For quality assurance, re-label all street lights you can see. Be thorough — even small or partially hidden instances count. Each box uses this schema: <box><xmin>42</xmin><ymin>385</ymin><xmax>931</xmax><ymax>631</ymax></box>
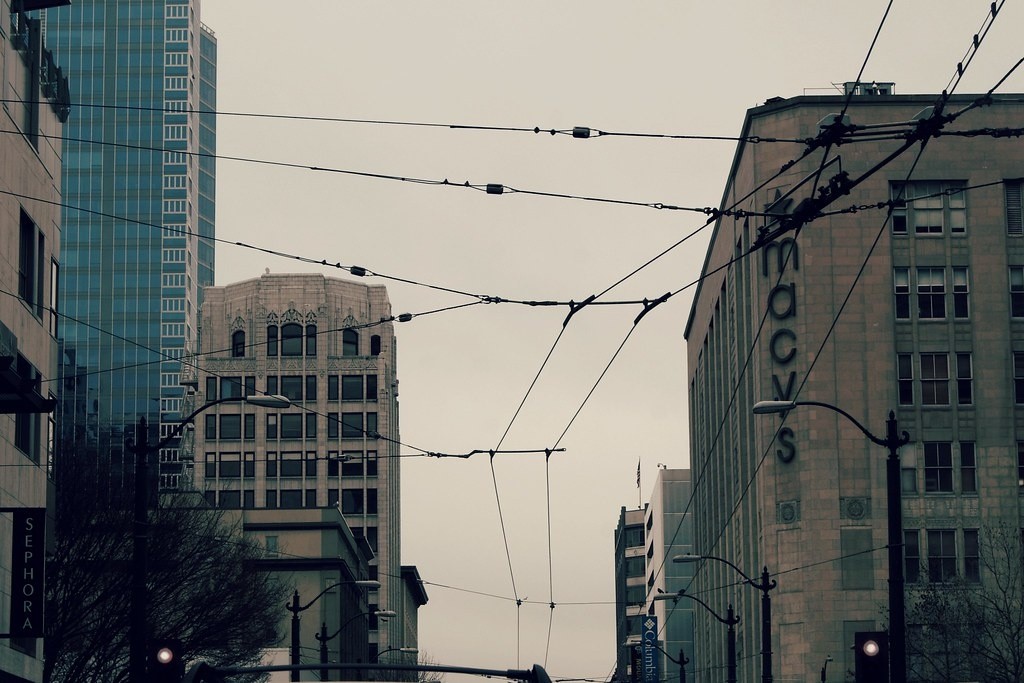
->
<box><xmin>671</xmin><ymin>555</ymin><xmax>774</xmax><ymax>683</ymax></box>
<box><xmin>321</xmin><ymin>611</ymin><xmax>396</xmax><ymax>682</ymax></box>
<box><xmin>654</xmin><ymin>593</ymin><xmax>737</xmax><ymax>683</ymax></box>
<box><xmin>132</xmin><ymin>393</ymin><xmax>291</xmax><ymax>683</ymax></box>
<box><xmin>752</xmin><ymin>399</ymin><xmax>907</xmax><ymax>683</ymax></box>
<box><xmin>631</xmin><ymin>640</ymin><xmax>686</xmax><ymax>683</ymax></box>
<box><xmin>361</xmin><ymin>647</ymin><xmax>420</xmax><ymax>683</ymax></box>
<box><xmin>290</xmin><ymin>580</ymin><xmax>381</xmax><ymax>682</ymax></box>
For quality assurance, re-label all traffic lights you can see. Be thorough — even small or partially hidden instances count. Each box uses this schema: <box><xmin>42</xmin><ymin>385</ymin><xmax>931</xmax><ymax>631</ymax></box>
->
<box><xmin>151</xmin><ymin>643</ymin><xmax>177</xmax><ymax>682</ymax></box>
<box><xmin>853</xmin><ymin>630</ymin><xmax>889</xmax><ymax>683</ymax></box>
